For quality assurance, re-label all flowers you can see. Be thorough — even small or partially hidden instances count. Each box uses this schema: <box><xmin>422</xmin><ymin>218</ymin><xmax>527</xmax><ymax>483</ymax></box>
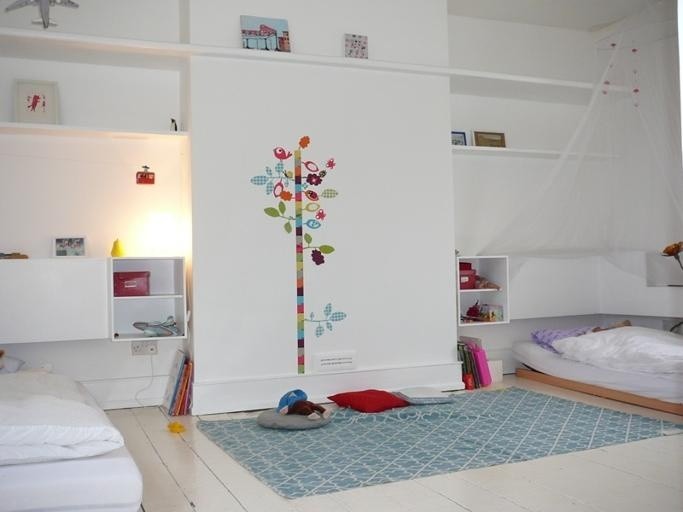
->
<box><xmin>659</xmin><ymin>241</ymin><xmax>683</xmax><ymax>273</ymax></box>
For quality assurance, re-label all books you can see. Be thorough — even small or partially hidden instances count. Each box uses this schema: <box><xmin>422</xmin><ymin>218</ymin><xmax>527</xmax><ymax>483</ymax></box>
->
<box><xmin>457</xmin><ymin>335</ymin><xmax>492</xmax><ymax>391</ymax></box>
<box><xmin>162</xmin><ymin>349</ymin><xmax>194</xmax><ymax>415</ymax></box>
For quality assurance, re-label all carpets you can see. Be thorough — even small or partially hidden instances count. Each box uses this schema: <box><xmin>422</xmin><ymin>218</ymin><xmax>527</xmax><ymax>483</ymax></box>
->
<box><xmin>195</xmin><ymin>384</ymin><xmax>682</xmax><ymax>501</ymax></box>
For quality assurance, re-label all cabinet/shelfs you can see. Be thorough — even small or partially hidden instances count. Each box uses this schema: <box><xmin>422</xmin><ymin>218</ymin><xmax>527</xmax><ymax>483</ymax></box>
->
<box><xmin>107</xmin><ymin>255</ymin><xmax>188</xmax><ymax>342</ymax></box>
<box><xmin>455</xmin><ymin>254</ymin><xmax>510</xmax><ymax>327</ymax></box>
<box><xmin>0</xmin><ymin>28</ymin><xmax>189</xmax><ymax>143</ymax></box>
<box><xmin>449</xmin><ymin>68</ymin><xmax>623</xmax><ymax>160</ymax></box>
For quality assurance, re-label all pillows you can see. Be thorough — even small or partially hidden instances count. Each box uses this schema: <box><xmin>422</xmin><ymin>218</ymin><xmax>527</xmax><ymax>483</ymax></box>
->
<box><xmin>531</xmin><ymin>326</ymin><xmax>594</xmax><ymax>354</ymax></box>
<box><xmin>256</xmin><ymin>385</ymin><xmax>453</xmax><ymax>431</ymax></box>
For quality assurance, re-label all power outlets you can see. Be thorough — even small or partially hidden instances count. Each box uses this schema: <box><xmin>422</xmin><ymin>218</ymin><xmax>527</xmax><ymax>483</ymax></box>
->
<box><xmin>130</xmin><ymin>341</ymin><xmax>158</xmax><ymax>355</ymax></box>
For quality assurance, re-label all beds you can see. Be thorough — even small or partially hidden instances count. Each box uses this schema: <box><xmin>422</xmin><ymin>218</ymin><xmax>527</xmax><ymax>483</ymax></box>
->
<box><xmin>513</xmin><ymin>340</ymin><xmax>682</xmax><ymax>414</ymax></box>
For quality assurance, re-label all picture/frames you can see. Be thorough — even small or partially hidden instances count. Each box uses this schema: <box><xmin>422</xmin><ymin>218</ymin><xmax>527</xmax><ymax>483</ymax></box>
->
<box><xmin>49</xmin><ymin>233</ymin><xmax>90</xmax><ymax>259</ymax></box>
<box><xmin>10</xmin><ymin>78</ymin><xmax>61</xmax><ymax>126</ymax></box>
<box><xmin>450</xmin><ymin>130</ymin><xmax>505</xmax><ymax>148</ymax></box>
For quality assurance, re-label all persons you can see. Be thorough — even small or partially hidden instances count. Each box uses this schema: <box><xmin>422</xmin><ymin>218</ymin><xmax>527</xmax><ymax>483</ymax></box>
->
<box><xmin>59</xmin><ymin>239</ymin><xmax>84</xmax><ymax>256</ymax></box>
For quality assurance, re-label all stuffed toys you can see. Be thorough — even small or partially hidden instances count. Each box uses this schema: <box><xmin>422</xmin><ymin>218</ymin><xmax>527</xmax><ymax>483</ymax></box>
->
<box><xmin>277</xmin><ymin>390</ymin><xmax>333</xmax><ymax>420</ymax></box>
